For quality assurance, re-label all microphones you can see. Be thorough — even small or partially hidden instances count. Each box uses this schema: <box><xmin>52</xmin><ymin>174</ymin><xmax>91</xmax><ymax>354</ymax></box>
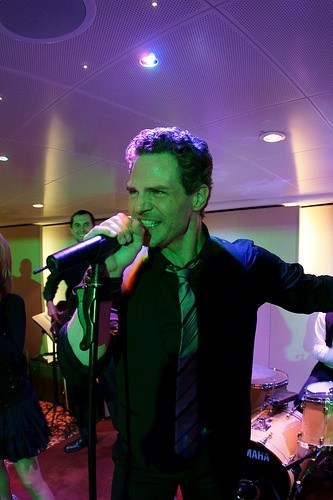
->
<box><xmin>33</xmin><ymin>229</ymin><xmax>120</xmax><ymax>276</ymax></box>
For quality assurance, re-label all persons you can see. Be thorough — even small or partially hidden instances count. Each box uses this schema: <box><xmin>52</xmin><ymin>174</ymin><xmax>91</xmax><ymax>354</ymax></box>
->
<box><xmin>43</xmin><ymin>209</ymin><xmax>96</xmax><ymax>453</ymax></box>
<box><xmin>83</xmin><ymin>126</ymin><xmax>333</xmax><ymax>500</ymax></box>
<box><xmin>0</xmin><ymin>233</ymin><xmax>55</xmax><ymax>500</ymax></box>
<box><xmin>294</xmin><ymin>312</ymin><xmax>333</xmax><ymax>414</ymax></box>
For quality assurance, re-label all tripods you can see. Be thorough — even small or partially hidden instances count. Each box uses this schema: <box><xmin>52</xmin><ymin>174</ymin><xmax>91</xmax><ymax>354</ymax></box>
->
<box><xmin>33</xmin><ymin>310</ymin><xmax>80</xmax><ymax>436</ymax></box>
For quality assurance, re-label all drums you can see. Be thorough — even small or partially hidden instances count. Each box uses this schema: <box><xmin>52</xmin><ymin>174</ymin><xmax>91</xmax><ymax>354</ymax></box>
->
<box><xmin>239</xmin><ymin>405</ymin><xmax>318</xmax><ymax>500</ymax></box>
<box><xmin>297</xmin><ymin>381</ymin><xmax>333</xmax><ymax>452</ymax></box>
<box><xmin>251</xmin><ymin>365</ymin><xmax>288</xmax><ymax>415</ymax></box>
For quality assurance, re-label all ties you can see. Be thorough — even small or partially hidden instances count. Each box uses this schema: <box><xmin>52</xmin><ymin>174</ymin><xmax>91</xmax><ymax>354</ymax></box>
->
<box><xmin>165</xmin><ymin>257</ymin><xmax>206</xmax><ymax>452</ymax></box>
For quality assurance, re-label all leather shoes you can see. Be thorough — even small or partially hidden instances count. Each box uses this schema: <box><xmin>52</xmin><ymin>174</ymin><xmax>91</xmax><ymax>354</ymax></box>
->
<box><xmin>64</xmin><ymin>437</ymin><xmax>87</xmax><ymax>452</ymax></box>
